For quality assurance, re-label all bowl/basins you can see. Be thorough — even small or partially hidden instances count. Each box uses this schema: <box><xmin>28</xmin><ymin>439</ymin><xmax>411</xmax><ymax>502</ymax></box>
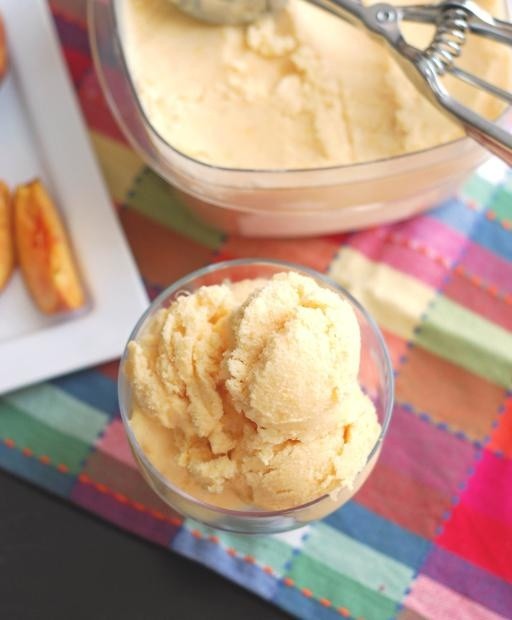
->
<box><xmin>84</xmin><ymin>1</ymin><xmax>512</xmax><ymax>245</ymax></box>
<box><xmin>118</xmin><ymin>256</ymin><xmax>394</xmax><ymax>534</ymax></box>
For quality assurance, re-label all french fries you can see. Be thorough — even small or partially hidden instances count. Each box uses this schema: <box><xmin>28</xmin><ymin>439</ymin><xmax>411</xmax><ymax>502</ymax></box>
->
<box><xmin>1</xmin><ymin>179</ymin><xmax>83</xmax><ymax>315</ymax></box>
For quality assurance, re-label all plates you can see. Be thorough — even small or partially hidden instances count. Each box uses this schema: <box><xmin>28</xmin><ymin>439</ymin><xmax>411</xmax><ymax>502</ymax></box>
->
<box><xmin>0</xmin><ymin>0</ymin><xmax>151</xmax><ymax>404</ymax></box>
